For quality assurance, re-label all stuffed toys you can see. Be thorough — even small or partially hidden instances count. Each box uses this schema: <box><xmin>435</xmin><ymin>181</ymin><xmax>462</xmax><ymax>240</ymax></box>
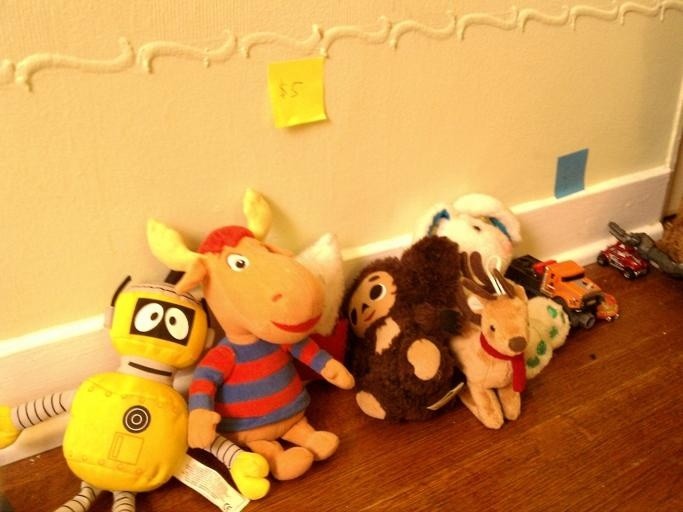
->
<box><xmin>145</xmin><ymin>188</ymin><xmax>356</xmax><ymax>479</ymax></box>
<box><xmin>0</xmin><ymin>187</ymin><xmax>572</xmax><ymax>512</ymax></box>
<box><xmin>1</xmin><ymin>270</ymin><xmax>271</xmax><ymax>512</ymax></box>
<box><xmin>339</xmin><ymin>192</ymin><xmax>570</xmax><ymax>429</ymax></box>
